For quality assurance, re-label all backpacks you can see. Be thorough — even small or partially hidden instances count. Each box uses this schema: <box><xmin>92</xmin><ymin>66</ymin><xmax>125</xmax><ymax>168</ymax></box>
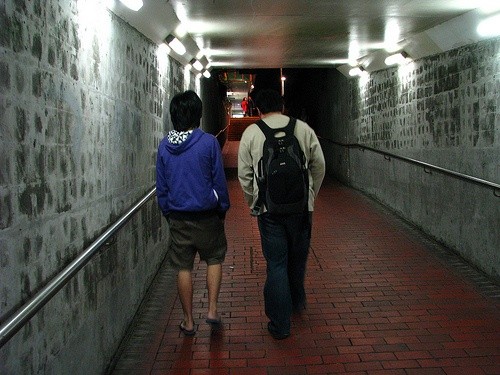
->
<box><xmin>250</xmin><ymin>117</ymin><xmax>308</xmax><ymax>216</ymax></box>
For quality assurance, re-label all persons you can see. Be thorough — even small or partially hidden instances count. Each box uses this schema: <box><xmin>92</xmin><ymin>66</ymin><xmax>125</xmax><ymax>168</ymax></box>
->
<box><xmin>238</xmin><ymin>89</ymin><xmax>325</xmax><ymax>339</ymax></box>
<box><xmin>241</xmin><ymin>96</ymin><xmax>254</xmax><ymax>117</ymax></box>
<box><xmin>155</xmin><ymin>90</ymin><xmax>231</xmax><ymax>337</ymax></box>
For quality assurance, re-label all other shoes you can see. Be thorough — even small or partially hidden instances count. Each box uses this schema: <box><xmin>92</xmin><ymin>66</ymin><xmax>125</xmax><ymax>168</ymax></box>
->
<box><xmin>268</xmin><ymin>323</ymin><xmax>289</xmax><ymax>339</ymax></box>
<box><xmin>178</xmin><ymin>321</ymin><xmax>194</xmax><ymax>336</ymax></box>
<box><xmin>205</xmin><ymin>319</ymin><xmax>219</xmax><ymax>325</ymax></box>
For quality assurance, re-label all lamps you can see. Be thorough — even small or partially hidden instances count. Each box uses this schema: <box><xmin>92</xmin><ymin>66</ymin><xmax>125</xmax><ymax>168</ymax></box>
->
<box><xmin>384</xmin><ymin>50</ymin><xmax>407</xmax><ymax>65</ymax></box>
<box><xmin>166</xmin><ymin>34</ymin><xmax>186</xmax><ymax>56</ymax></box>
<box><xmin>349</xmin><ymin>66</ymin><xmax>364</xmax><ymax>76</ymax></box>
<box><xmin>190</xmin><ymin>58</ymin><xmax>204</xmax><ymax>72</ymax></box>
